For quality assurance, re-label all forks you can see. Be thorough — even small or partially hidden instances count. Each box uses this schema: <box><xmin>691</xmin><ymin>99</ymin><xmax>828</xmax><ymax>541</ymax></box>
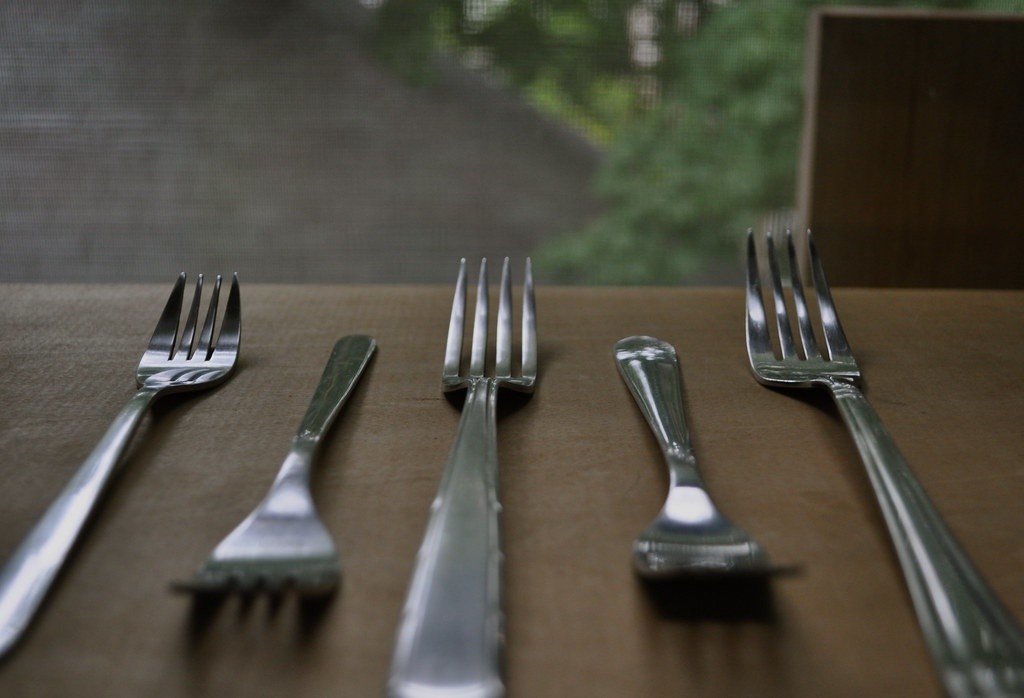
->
<box><xmin>613</xmin><ymin>334</ymin><xmax>806</xmax><ymax>594</ymax></box>
<box><xmin>168</xmin><ymin>332</ymin><xmax>380</xmax><ymax>609</ymax></box>
<box><xmin>383</xmin><ymin>256</ymin><xmax>538</xmax><ymax>698</ymax></box>
<box><xmin>0</xmin><ymin>271</ymin><xmax>241</xmax><ymax>666</ymax></box>
<box><xmin>744</xmin><ymin>227</ymin><xmax>1024</xmax><ymax>698</ymax></box>
